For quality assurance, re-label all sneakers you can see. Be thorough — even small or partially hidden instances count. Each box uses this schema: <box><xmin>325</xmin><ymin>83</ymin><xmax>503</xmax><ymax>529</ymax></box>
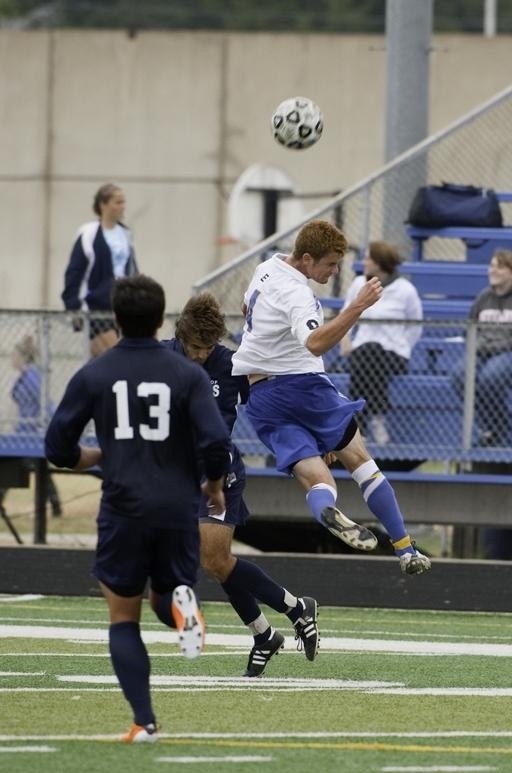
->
<box><xmin>292</xmin><ymin>596</ymin><xmax>320</xmax><ymax>662</ymax></box>
<box><xmin>245</xmin><ymin>626</ymin><xmax>286</xmax><ymax>677</ymax></box>
<box><xmin>320</xmin><ymin>506</ymin><xmax>378</xmax><ymax>551</ymax></box>
<box><xmin>400</xmin><ymin>543</ymin><xmax>432</xmax><ymax>576</ymax></box>
<box><xmin>371</xmin><ymin>417</ymin><xmax>389</xmax><ymax>444</ymax></box>
<box><xmin>172</xmin><ymin>584</ymin><xmax>206</xmax><ymax>659</ymax></box>
<box><xmin>122</xmin><ymin>723</ymin><xmax>161</xmax><ymax>744</ymax></box>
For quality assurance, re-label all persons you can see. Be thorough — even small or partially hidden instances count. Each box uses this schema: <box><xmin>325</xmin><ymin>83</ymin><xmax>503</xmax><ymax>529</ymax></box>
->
<box><xmin>155</xmin><ymin>291</ymin><xmax>319</xmax><ymax>679</ymax></box>
<box><xmin>0</xmin><ymin>335</ymin><xmax>62</xmax><ymax>521</ymax></box>
<box><xmin>449</xmin><ymin>249</ymin><xmax>511</xmax><ymax>446</ymax></box>
<box><xmin>338</xmin><ymin>241</ymin><xmax>422</xmax><ymax>446</ymax></box>
<box><xmin>59</xmin><ymin>184</ymin><xmax>141</xmax><ymax>440</ymax></box>
<box><xmin>229</xmin><ymin>215</ymin><xmax>435</xmax><ymax>578</ymax></box>
<box><xmin>45</xmin><ymin>270</ymin><xmax>237</xmax><ymax>746</ymax></box>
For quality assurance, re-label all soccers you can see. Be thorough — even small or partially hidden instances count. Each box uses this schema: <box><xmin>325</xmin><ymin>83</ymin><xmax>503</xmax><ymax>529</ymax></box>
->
<box><xmin>269</xmin><ymin>96</ymin><xmax>326</xmax><ymax>150</ymax></box>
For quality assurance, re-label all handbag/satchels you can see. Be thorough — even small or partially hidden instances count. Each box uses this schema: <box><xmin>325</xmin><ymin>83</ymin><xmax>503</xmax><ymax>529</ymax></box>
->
<box><xmin>408</xmin><ymin>182</ymin><xmax>502</xmax><ymax>228</ymax></box>
